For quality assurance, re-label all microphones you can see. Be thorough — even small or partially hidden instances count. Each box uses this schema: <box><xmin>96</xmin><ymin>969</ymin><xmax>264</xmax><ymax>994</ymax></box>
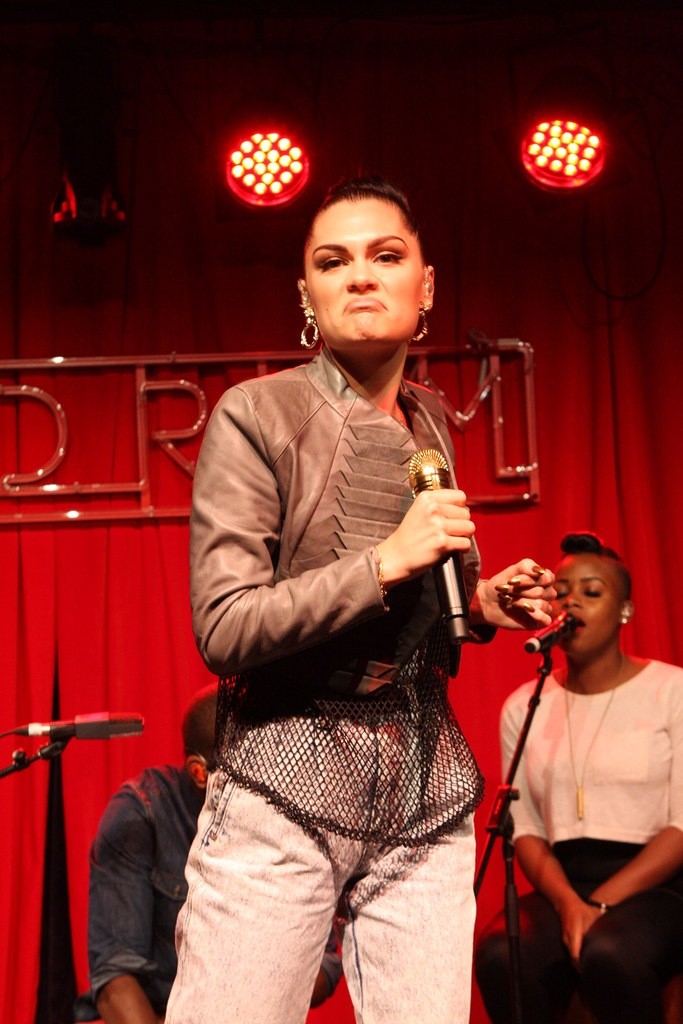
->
<box><xmin>410</xmin><ymin>449</ymin><xmax>471</xmax><ymax>643</ymax></box>
<box><xmin>524</xmin><ymin>611</ymin><xmax>575</xmax><ymax>653</ymax></box>
<box><xmin>13</xmin><ymin>712</ymin><xmax>144</xmax><ymax>739</ymax></box>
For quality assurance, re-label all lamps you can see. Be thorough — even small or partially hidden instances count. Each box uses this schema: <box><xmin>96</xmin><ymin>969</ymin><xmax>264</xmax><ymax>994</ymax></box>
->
<box><xmin>512</xmin><ymin>62</ymin><xmax>615</xmax><ymax>195</ymax></box>
<box><xmin>221</xmin><ymin>95</ymin><xmax>311</xmax><ymax>211</ymax></box>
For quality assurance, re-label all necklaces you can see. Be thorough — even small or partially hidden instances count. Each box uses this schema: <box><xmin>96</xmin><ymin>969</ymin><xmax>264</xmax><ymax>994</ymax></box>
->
<box><xmin>563</xmin><ymin>651</ymin><xmax>625</xmax><ymax>819</ymax></box>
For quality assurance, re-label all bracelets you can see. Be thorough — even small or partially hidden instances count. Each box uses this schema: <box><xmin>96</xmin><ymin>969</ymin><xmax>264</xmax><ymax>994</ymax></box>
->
<box><xmin>587</xmin><ymin>896</ymin><xmax>615</xmax><ymax>913</ymax></box>
<box><xmin>370</xmin><ymin>544</ymin><xmax>387</xmax><ymax>597</ymax></box>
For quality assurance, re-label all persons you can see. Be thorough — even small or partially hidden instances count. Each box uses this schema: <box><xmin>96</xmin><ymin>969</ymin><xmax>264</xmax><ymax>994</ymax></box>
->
<box><xmin>89</xmin><ymin>683</ymin><xmax>342</xmax><ymax>1024</ymax></box>
<box><xmin>164</xmin><ymin>176</ymin><xmax>558</xmax><ymax>1024</ymax></box>
<box><xmin>474</xmin><ymin>533</ymin><xmax>683</xmax><ymax>1024</ymax></box>
